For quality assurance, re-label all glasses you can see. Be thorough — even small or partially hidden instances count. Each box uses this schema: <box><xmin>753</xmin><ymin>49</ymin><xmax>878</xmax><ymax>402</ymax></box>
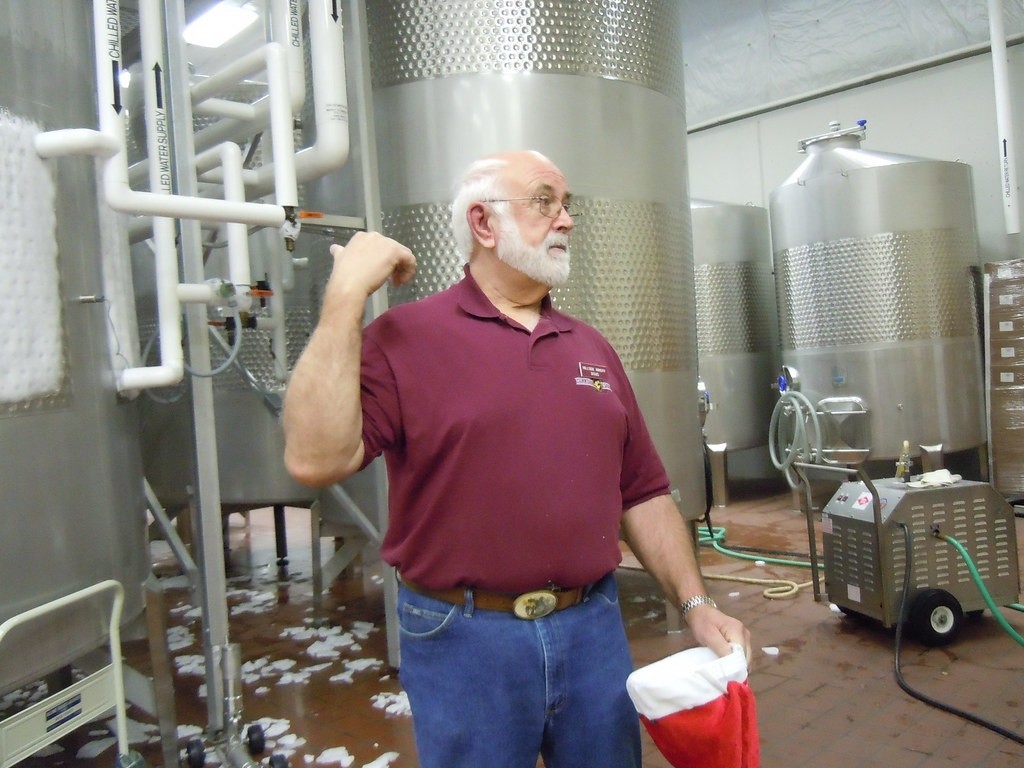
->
<box><xmin>483</xmin><ymin>193</ymin><xmax>582</xmax><ymax>219</ymax></box>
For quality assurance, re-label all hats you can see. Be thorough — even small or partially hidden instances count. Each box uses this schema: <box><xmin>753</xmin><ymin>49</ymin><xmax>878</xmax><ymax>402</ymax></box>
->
<box><xmin>626</xmin><ymin>644</ymin><xmax>759</xmax><ymax>768</ymax></box>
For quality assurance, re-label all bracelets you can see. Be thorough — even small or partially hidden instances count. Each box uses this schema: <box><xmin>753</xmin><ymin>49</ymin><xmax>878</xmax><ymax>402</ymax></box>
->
<box><xmin>682</xmin><ymin>594</ymin><xmax>717</xmax><ymax>625</ymax></box>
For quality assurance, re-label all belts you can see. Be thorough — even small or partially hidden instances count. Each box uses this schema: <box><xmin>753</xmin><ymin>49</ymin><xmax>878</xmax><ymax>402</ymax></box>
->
<box><xmin>399</xmin><ymin>573</ymin><xmax>584</xmax><ymax>619</ymax></box>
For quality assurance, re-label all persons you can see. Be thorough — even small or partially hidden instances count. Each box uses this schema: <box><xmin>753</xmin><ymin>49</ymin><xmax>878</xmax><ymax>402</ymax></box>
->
<box><xmin>282</xmin><ymin>151</ymin><xmax>754</xmax><ymax>768</ymax></box>
